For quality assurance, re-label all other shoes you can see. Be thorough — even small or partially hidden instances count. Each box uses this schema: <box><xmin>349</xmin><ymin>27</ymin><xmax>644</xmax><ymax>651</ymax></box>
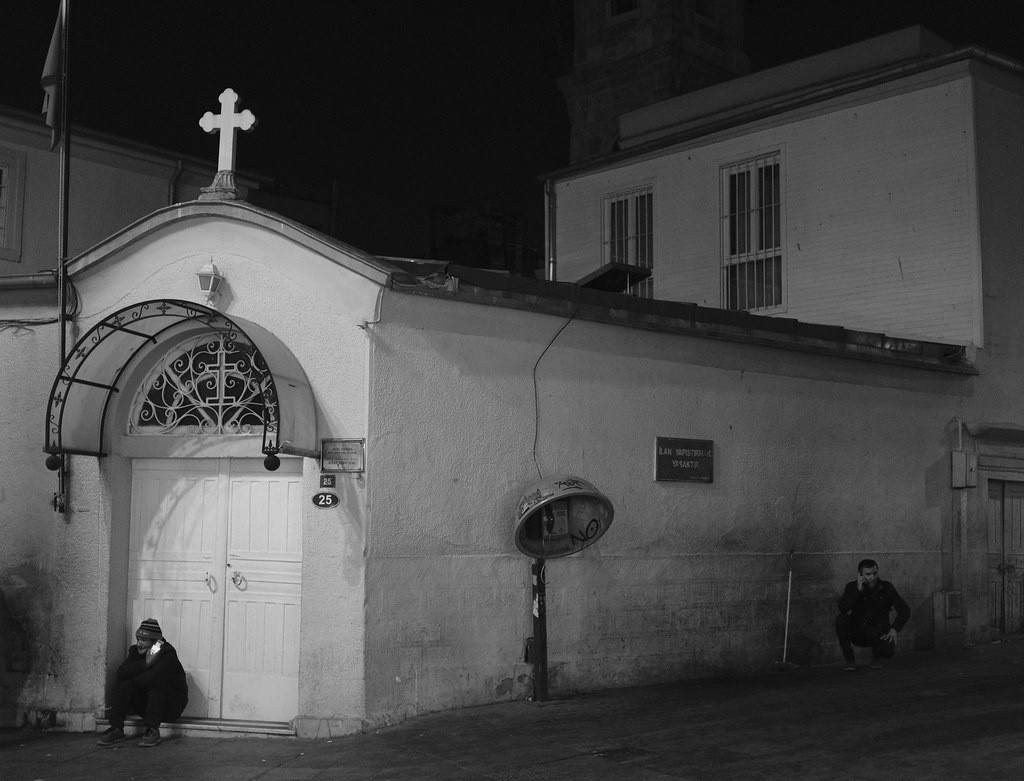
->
<box><xmin>844</xmin><ymin>655</ymin><xmax>855</xmax><ymax>670</ymax></box>
<box><xmin>871</xmin><ymin>650</ymin><xmax>882</xmax><ymax>669</ymax></box>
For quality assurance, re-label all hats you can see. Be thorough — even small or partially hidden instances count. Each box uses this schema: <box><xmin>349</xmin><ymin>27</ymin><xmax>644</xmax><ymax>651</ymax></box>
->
<box><xmin>135</xmin><ymin>618</ymin><xmax>162</xmax><ymax>639</ymax></box>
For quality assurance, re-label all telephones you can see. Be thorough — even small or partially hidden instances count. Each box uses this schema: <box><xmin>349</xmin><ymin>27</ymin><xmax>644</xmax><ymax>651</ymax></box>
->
<box><xmin>532</xmin><ymin>501</ymin><xmax>570</xmax><ymax>541</ymax></box>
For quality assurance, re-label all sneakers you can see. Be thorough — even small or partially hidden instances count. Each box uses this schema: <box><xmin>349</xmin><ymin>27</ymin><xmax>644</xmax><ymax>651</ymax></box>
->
<box><xmin>97</xmin><ymin>727</ymin><xmax>125</xmax><ymax>745</ymax></box>
<box><xmin>138</xmin><ymin>727</ymin><xmax>161</xmax><ymax>747</ymax></box>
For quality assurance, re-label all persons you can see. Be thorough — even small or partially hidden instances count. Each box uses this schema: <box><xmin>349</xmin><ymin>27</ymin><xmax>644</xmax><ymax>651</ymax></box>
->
<box><xmin>835</xmin><ymin>559</ymin><xmax>910</xmax><ymax>670</ymax></box>
<box><xmin>96</xmin><ymin>618</ymin><xmax>189</xmax><ymax>747</ymax></box>
